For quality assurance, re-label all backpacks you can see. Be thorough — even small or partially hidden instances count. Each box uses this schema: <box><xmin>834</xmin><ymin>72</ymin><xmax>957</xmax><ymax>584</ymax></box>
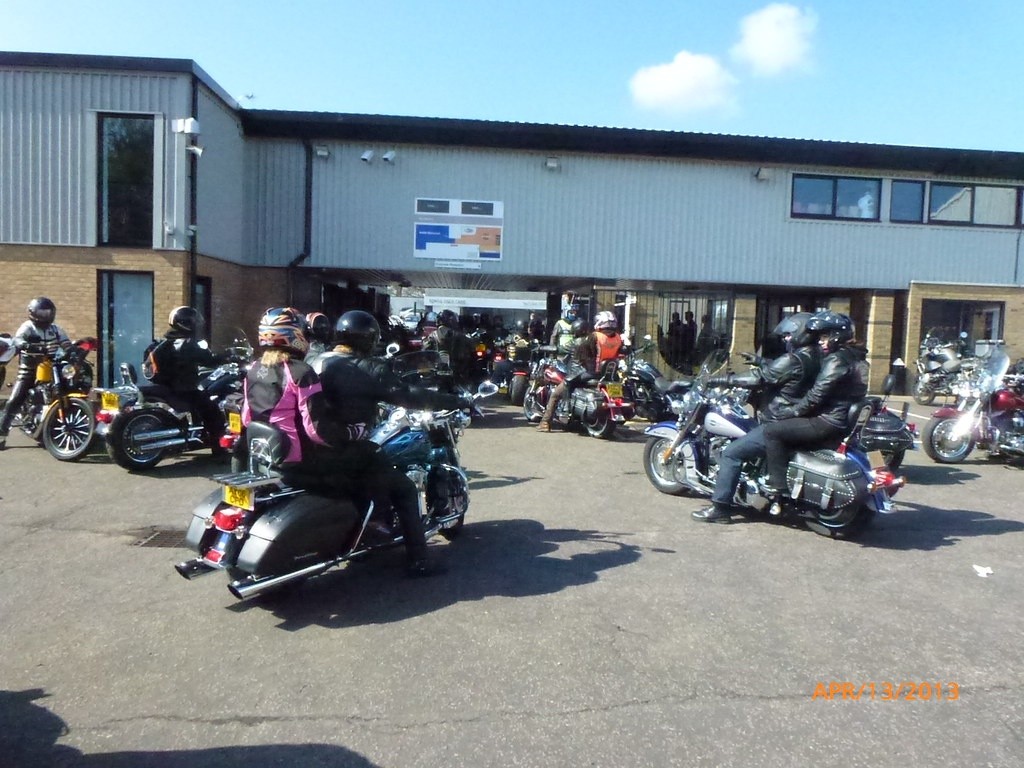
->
<box><xmin>141</xmin><ymin>336</ymin><xmax>179</xmax><ymax>384</ymax></box>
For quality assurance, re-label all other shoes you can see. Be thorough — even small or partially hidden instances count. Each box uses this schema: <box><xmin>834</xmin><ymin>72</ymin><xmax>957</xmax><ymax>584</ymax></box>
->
<box><xmin>760</xmin><ymin>483</ymin><xmax>786</xmax><ymax>496</ymax></box>
<box><xmin>555</xmin><ymin>407</ymin><xmax>569</xmax><ymax>415</ymax></box>
<box><xmin>211</xmin><ymin>452</ymin><xmax>233</xmax><ymax>464</ymax></box>
<box><xmin>406</xmin><ymin>558</ymin><xmax>448</xmax><ymax>578</ymax></box>
<box><xmin>535</xmin><ymin>420</ymin><xmax>549</xmax><ymax>432</ymax></box>
<box><xmin>361</xmin><ymin>520</ymin><xmax>391</xmax><ymax>540</ymax></box>
<box><xmin>0</xmin><ymin>435</ymin><xmax>6</xmax><ymax>450</ymax></box>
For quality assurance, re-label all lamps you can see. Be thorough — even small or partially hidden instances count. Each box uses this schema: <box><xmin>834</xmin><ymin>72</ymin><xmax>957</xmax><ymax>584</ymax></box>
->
<box><xmin>315</xmin><ymin>145</ymin><xmax>330</xmax><ymax>159</ymax></box>
<box><xmin>183</xmin><ymin>116</ymin><xmax>200</xmax><ymax>137</ymax></box>
<box><xmin>755</xmin><ymin>167</ymin><xmax>770</xmax><ymax>180</ymax></box>
<box><xmin>545</xmin><ymin>155</ymin><xmax>562</xmax><ymax>170</ymax></box>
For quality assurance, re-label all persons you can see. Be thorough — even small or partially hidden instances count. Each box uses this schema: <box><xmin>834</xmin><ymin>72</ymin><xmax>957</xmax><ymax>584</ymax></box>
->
<box><xmin>492</xmin><ymin>320</ymin><xmax>531</xmax><ymax>384</ymax></box>
<box><xmin>683</xmin><ymin>311</ymin><xmax>697</xmax><ymax>375</ymax></box>
<box><xmin>549</xmin><ymin>305</ymin><xmax>579</xmax><ymax>360</ymax></box>
<box><xmin>311</xmin><ymin>310</ymin><xmax>477</xmax><ymax>578</ymax></box>
<box><xmin>532</xmin><ymin>318</ymin><xmax>590</xmax><ymax>431</ymax></box>
<box><xmin>690</xmin><ymin>312</ymin><xmax>823</xmax><ymax>524</ymax></box>
<box><xmin>528</xmin><ymin>313</ymin><xmax>548</xmax><ymax>345</ymax></box>
<box><xmin>697</xmin><ymin>314</ymin><xmax>713</xmax><ymax>360</ymax></box>
<box><xmin>760</xmin><ymin>310</ymin><xmax>870</xmax><ymax>495</ymax></box>
<box><xmin>658</xmin><ymin>324</ymin><xmax>670</xmax><ymax>361</ymax></box>
<box><xmin>667</xmin><ymin>311</ymin><xmax>683</xmax><ymax>369</ymax></box>
<box><xmin>458</xmin><ymin>313</ymin><xmax>512</xmax><ymax>368</ymax></box>
<box><xmin>0</xmin><ymin>296</ymin><xmax>75</xmax><ymax>450</ymax></box>
<box><xmin>556</xmin><ymin>310</ymin><xmax>623</xmax><ymax>417</ymax></box>
<box><xmin>164</xmin><ymin>305</ymin><xmax>233</xmax><ymax>466</ymax></box>
<box><xmin>240</xmin><ymin>306</ymin><xmax>391</xmax><ymax>540</ymax></box>
<box><xmin>304</xmin><ymin>312</ymin><xmax>329</xmax><ymax>364</ymax></box>
<box><xmin>421</xmin><ymin>309</ymin><xmax>477</xmax><ymax>384</ymax></box>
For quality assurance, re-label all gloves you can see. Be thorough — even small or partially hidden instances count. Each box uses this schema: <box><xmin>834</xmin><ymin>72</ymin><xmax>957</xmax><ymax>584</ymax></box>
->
<box><xmin>347</xmin><ymin>423</ymin><xmax>371</xmax><ymax>441</ymax></box>
<box><xmin>23</xmin><ymin>343</ymin><xmax>37</xmax><ymax>352</ymax></box>
<box><xmin>462</xmin><ymin>395</ymin><xmax>475</xmax><ymax>414</ymax></box>
<box><xmin>737</xmin><ymin>352</ymin><xmax>761</xmax><ymax>366</ymax></box>
<box><xmin>774</xmin><ymin>405</ymin><xmax>796</xmax><ymax>420</ymax></box>
<box><xmin>68</xmin><ymin>346</ymin><xmax>78</xmax><ymax>353</ymax></box>
<box><xmin>702</xmin><ymin>375</ymin><xmax>722</xmax><ymax>388</ymax></box>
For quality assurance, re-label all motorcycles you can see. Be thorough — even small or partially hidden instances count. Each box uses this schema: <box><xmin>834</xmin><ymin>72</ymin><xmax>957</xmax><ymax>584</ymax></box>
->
<box><xmin>219</xmin><ymin>344</ymin><xmax>401</xmax><ymax>475</ymax></box>
<box><xmin>643</xmin><ymin>350</ymin><xmax>921</xmax><ymax>541</ymax></box>
<box><xmin>11</xmin><ymin>343</ymin><xmax>97</xmax><ymax>462</ymax></box>
<box><xmin>466</xmin><ymin>312</ymin><xmax>635</xmax><ymax>438</ymax></box>
<box><xmin>173</xmin><ymin>351</ymin><xmax>500</xmax><ymax>600</ymax></box>
<box><xmin>913</xmin><ymin>327</ymin><xmax>1024</xmax><ymax>464</ymax></box>
<box><xmin>97</xmin><ymin>328</ymin><xmax>254</xmax><ymax>470</ymax></box>
<box><xmin>618</xmin><ymin>348</ymin><xmax>690</xmax><ymax>423</ymax></box>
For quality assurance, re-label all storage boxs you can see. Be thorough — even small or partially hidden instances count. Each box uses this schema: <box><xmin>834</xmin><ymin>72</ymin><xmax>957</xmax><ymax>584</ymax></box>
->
<box><xmin>235</xmin><ymin>486</ymin><xmax>359</xmax><ymax>573</ymax></box>
<box><xmin>570</xmin><ymin>387</ymin><xmax>606</xmax><ymax>422</ymax></box>
<box><xmin>785</xmin><ymin>451</ymin><xmax>870</xmax><ymax>510</ymax></box>
<box><xmin>859</xmin><ymin>408</ymin><xmax>914</xmax><ymax>454</ymax></box>
<box><xmin>186</xmin><ymin>485</ymin><xmax>229</xmax><ymax>555</ymax></box>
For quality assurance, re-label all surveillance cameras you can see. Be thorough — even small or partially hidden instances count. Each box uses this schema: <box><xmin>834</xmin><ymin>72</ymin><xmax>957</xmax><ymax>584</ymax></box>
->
<box><xmin>382</xmin><ymin>151</ymin><xmax>396</xmax><ymax>162</ymax></box>
<box><xmin>361</xmin><ymin>150</ymin><xmax>374</xmax><ymax>162</ymax></box>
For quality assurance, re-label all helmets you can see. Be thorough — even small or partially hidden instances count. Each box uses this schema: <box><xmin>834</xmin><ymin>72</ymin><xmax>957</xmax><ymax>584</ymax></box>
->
<box><xmin>27</xmin><ymin>297</ymin><xmax>56</xmax><ymax>329</ymax></box>
<box><xmin>333</xmin><ymin>310</ymin><xmax>380</xmax><ymax>359</ymax></box>
<box><xmin>168</xmin><ymin>306</ymin><xmax>205</xmax><ymax>340</ymax></box>
<box><xmin>783</xmin><ymin>312</ymin><xmax>816</xmax><ymax>350</ymax></box>
<box><xmin>258</xmin><ymin>307</ymin><xmax>311</xmax><ymax>359</ymax></box>
<box><xmin>804</xmin><ymin>309</ymin><xmax>855</xmax><ymax>353</ymax></box>
<box><xmin>306</xmin><ymin>313</ymin><xmax>330</xmax><ymax>339</ymax></box>
<box><xmin>418</xmin><ymin>302</ymin><xmax>618</xmax><ymax>332</ymax></box>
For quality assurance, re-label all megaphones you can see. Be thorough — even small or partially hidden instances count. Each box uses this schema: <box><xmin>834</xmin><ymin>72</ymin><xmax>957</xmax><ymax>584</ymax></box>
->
<box><xmin>187</xmin><ymin>144</ymin><xmax>204</xmax><ymax>157</ymax></box>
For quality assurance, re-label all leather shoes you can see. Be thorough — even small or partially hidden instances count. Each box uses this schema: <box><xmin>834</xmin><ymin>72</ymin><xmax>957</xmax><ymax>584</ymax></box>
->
<box><xmin>692</xmin><ymin>506</ymin><xmax>731</xmax><ymax>524</ymax></box>
